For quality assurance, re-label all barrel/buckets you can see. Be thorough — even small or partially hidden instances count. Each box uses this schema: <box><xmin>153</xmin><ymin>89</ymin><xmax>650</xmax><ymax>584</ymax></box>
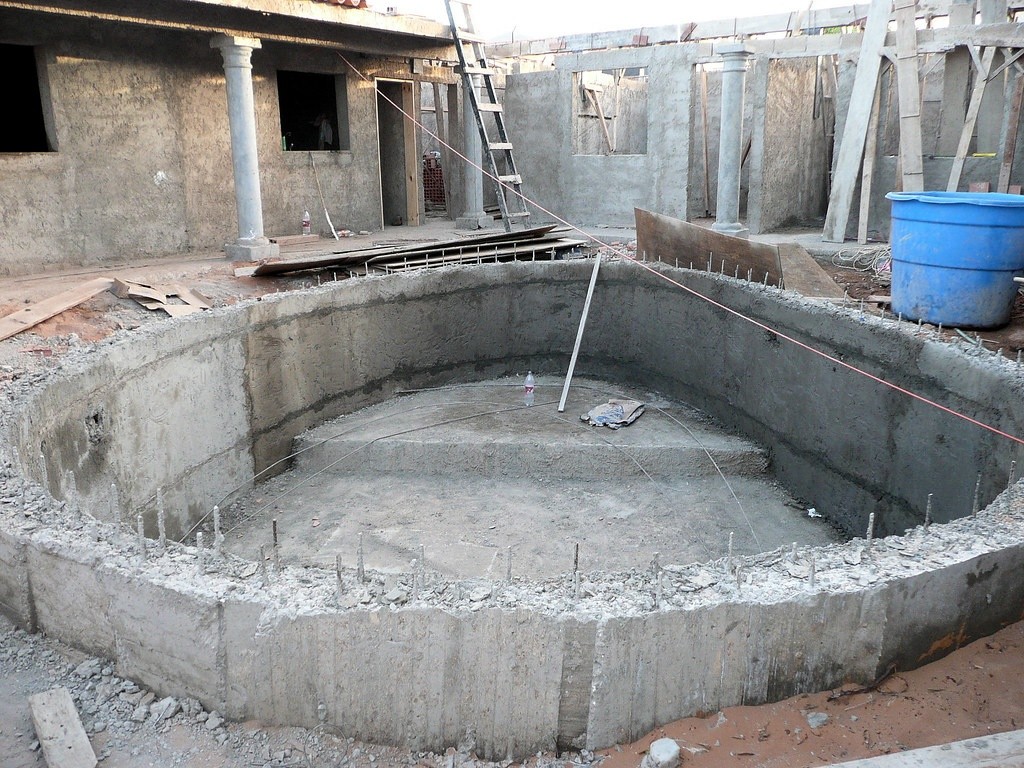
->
<box><xmin>885</xmin><ymin>191</ymin><xmax>1024</xmax><ymax>326</ymax></box>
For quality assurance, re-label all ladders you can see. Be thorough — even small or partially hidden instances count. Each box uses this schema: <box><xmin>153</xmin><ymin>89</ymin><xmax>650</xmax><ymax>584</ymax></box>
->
<box><xmin>444</xmin><ymin>0</ymin><xmax>532</xmax><ymax>233</ymax></box>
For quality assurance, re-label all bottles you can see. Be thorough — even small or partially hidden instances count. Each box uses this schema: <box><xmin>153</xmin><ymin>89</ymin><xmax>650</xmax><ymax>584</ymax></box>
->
<box><xmin>525</xmin><ymin>371</ymin><xmax>535</xmax><ymax>407</ymax></box>
<box><xmin>302</xmin><ymin>209</ymin><xmax>310</xmax><ymax>235</ymax></box>
